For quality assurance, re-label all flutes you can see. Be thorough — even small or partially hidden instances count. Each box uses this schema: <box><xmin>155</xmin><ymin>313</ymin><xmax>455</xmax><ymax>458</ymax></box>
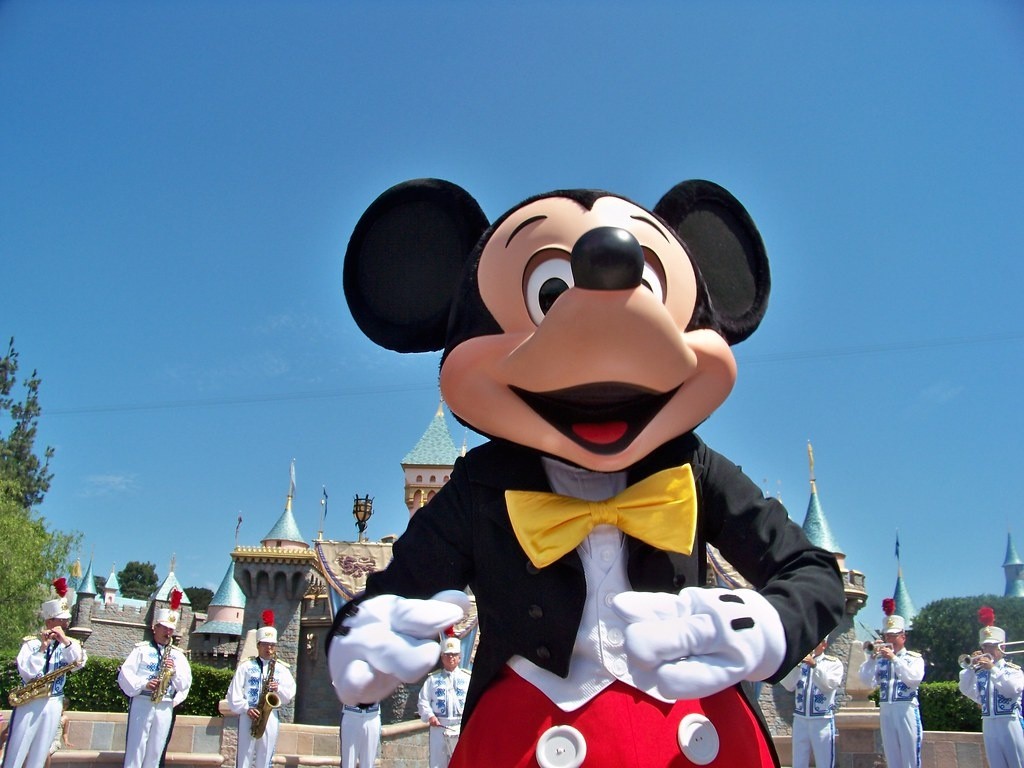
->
<box><xmin>47</xmin><ymin>627</ymin><xmax>71</xmax><ymax>635</ymax></box>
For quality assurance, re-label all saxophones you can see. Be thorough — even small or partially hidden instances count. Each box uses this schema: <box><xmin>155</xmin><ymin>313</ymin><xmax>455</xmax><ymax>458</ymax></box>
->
<box><xmin>150</xmin><ymin>634</ymin><xmax>176</xmax><ymax>704</ymax></box>
<box><xmin>250</xmin><ymin>650</ymin><xmax>282</xmax><ymax>739</ymax></box>
<box><xmin>7</xmin><ymin>639</ymin><xmax>84</xmax><ymax>707</ymax></box>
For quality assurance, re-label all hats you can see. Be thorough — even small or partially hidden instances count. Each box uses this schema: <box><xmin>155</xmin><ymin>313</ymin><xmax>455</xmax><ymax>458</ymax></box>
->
<box><xmin>441</xmin><ymin>625</ymin><xmax>462</xmax><ymax>654</ymax></box>
<box><xmin>881</xmin><ymin>598</ymin><xmax>906</xmax><ymax>635</ymax></box>
<box><xmin>43</xmin><ymin>578</ymin><xmax>72</xmax><ymax>621</ymax></box>
<box><xmin>978</xmin><ymin>608</ymin><xmax>1006</xmax><ymax>645</ymax></box>
<box><xmin>152</xmin><ymin>589</ymin><xmax>182</xmax><ymax>632</ymax></box>
<box><xmin>256</xmin><ymin>609</ymin><xmax>278</xmax><ymax>645</ymax></box>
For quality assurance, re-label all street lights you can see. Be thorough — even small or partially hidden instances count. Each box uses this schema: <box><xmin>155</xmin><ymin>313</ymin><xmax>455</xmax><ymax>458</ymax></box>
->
<box><xmin>352</xmin><ymin>493</ymin><xmax>376</xmax><ymax>542</ymax></box>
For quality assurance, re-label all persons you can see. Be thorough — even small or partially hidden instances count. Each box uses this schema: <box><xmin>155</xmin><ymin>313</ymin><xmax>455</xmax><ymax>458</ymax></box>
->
<box><xmin>858</xmin><ymin>615</ymin><xmax>926</xmax><ymax>768</ymax></box>
<box><xmin>958</xmin><ymin>625</ymin><xmax>1024</xmax><ymax>768</ymax></box>
<box><xmin>417</xmin><ymin>637</ymin><xmax>473</xmax><ymax>768</ymax></box>
<box><xmin>338</xmin><ymin>702</ymin><xmax>383</xmax><ymax>768</ymax></box>
<box><xmin>0</xmin><ymin>596</ymin><xmax>88</xmax><ymax>768</ymax></box>
<box><xmin>226</xmin><ymin>626</ymin><xmax>297</xmax><ymax>768</ymax></box>
<box><xmin>780</xmin><ymin>632</ymin><xmax>844</xmax><ymax>768</ymax></box>
<box><xmin>117</xmin><ymin>608</ymin><xmax>193</xmax><ymax>768</ymax></box>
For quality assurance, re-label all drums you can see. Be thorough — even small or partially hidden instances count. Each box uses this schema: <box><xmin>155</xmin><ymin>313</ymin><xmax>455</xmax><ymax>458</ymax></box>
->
<box><xmin>443</xmin><ymin>721</ymin><xmax>460</xmax><ymax>759</ymax></box>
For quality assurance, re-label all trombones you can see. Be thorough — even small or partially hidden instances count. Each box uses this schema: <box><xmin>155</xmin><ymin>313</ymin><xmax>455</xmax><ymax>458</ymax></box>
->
<box><xmin>997</xmin><ymin>640</ymin><xmax>1024</xmax><ymax>655</ymax></box>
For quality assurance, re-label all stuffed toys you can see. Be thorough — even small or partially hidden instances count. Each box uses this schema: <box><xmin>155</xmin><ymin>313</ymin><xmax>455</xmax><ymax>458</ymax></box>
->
<box><xmin>323</xmin><ymin>177</ymin><xmax>847</xmax><ymax>768</ymax></box>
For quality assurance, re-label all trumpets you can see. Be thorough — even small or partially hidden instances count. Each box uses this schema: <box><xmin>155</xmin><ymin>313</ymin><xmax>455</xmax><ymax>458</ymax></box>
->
<box><xmin>863</xmin><ymin>640</ymin><xmax>895</xmax><ymax>656</ymax></box>
<box><xmin>797</xmin><ymin>649</ymin><xmax>815</xmax><ymax>667</ymax></box>
<box><xmin>958</xmin><ymin>653</ymin><xmax>995</xmax><ymax>670</ymax></box>
